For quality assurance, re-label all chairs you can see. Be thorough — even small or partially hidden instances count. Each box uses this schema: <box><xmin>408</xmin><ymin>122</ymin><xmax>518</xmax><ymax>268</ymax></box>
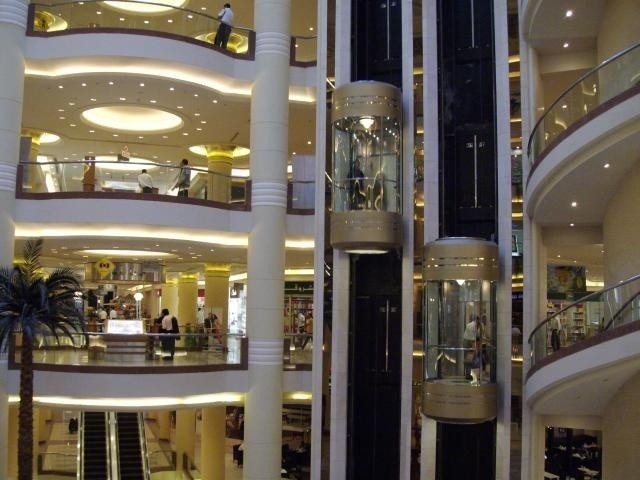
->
<box><xmin>88</xmin><ymin>334</ymin><xmax>108</xmax><ymax>361</ymax></box>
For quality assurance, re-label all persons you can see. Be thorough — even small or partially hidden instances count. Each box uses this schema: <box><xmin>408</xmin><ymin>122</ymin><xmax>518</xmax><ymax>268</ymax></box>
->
<box><xmin>348</xmin><ymin>159</ymin><xmax>386</xmax><ymax>210</ymax></box>
<box><xmin>463</xmin><ymin>315</ymin><xmax>489</xmax><ymax>380</ymax></box>
<box><xmin>110</xmin><ymin>307</ymin><xmax>118</xmax><ymax>320</ymax></box>
<box><xmin>175</xmin><ymin>158</ymin><xmax>191</xmax><ymax>197</ymax></box>
<box><xmin>137</xmin><ymin>169</ymin><xmax>153</xmax><ymax>193</ymax></box>
<box><xmin>550</xmin><ymin>312</ymin><xmax>561</xmax><ymax>352</ymax></box>
<box><xmin>214</xmin><ymin>3</ymin><xmax>234</xmax><ymax>49</ymax></box>
<box><xmin>204</xmin><ymin>312</ymin><xmax>223</xmax><ymax>344</ymax></box>
<box><xmin>295</xmin><ymin>313</ymin><xmax>313</xmax><ymax>350</ymax></box>
<box><xmin>155</xmin><ymin>309</ymin><xmax>180</xmax><ymax>358</ymax></box>
<box><xmin>99</xmin><ymin>307</ymin><xmax>108</xmax><ymax>322</ymax></box>
